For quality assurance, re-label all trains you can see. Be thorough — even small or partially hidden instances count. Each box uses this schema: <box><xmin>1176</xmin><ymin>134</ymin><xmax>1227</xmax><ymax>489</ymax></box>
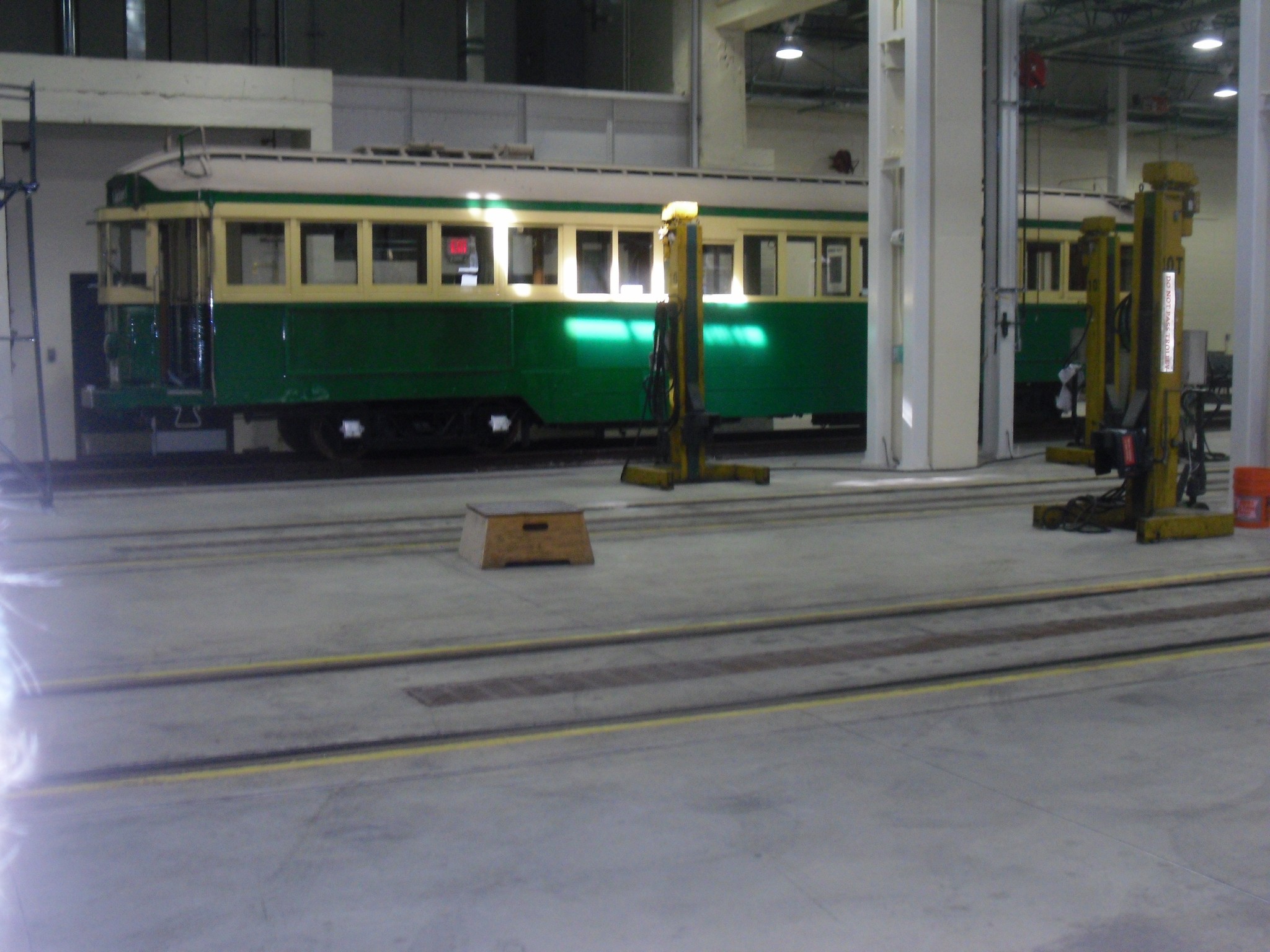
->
<box><xmin>71</xmin><ymin>122</ymin><xmax>1139</xmax><ymax>472</ymax></box>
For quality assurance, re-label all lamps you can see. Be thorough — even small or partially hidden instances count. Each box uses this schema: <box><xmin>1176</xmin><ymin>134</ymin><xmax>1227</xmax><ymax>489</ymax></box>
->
<box><xmin>773</xmin><ymin>13</ymin><xmax>807</xmax><ymax>59</ymax></box>
<box><xmin>1211</xmin><ymin>57</ymin><xmax>1239</xmax><ymax>98</ymax></box>
<box><xmin>1191</xmin><ymin>10</ymin><xmax>1226</xmax><ymax>51</ymax></box>
